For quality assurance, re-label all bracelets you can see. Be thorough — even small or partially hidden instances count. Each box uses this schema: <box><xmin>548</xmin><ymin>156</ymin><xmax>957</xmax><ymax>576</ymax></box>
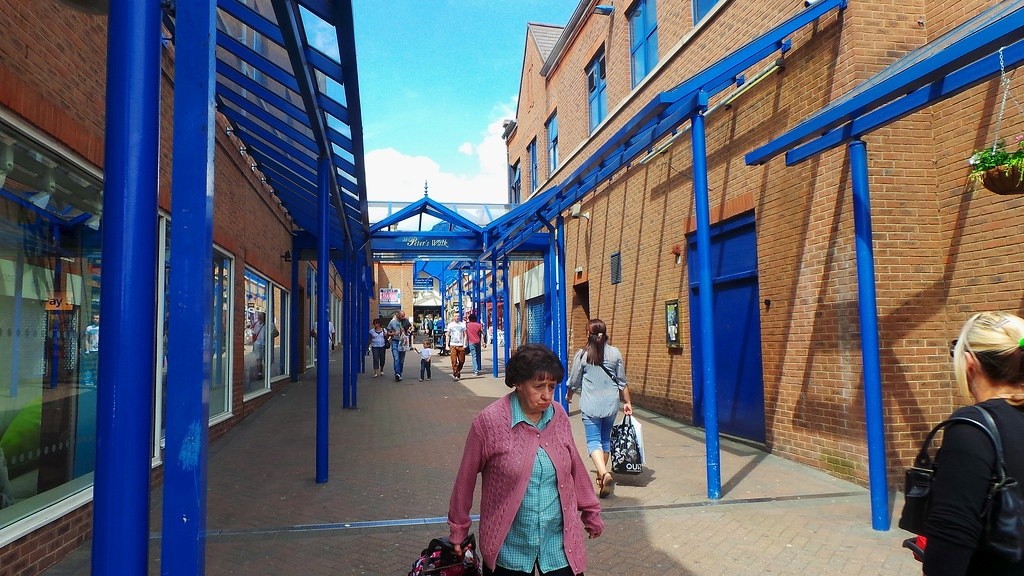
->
<box><xmin>566</xmin><ymin>394</ymin><xmax>572</xmax><ymax>399</ymax></box>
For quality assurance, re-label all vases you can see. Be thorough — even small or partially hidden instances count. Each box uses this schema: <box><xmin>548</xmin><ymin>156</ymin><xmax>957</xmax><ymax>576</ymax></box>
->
<box><xmin>978</xmin><ymin>164</ymin><xmax>1024</xmax><ymax>195</ymax></box>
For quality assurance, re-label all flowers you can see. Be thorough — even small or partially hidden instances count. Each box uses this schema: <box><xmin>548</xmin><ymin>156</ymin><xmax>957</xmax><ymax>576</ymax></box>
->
<box><xmin>967</xmin><ymin>132</ymin><xmax>1024</xmax><ymax>188</ymax></box>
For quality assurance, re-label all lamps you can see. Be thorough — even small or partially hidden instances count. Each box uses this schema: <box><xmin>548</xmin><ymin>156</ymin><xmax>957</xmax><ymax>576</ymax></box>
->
<box><xmin>591</xmin><ymin>4</ymin><xmax>614</xmax><ymax>16</ymax></box>
<box><xmin>280</xmin><ymin>250</ymin><xmax>293</xmax><ymax>263</ymax></box>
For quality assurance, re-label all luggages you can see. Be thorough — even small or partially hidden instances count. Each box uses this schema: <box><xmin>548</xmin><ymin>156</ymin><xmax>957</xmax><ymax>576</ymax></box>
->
<box><xmin>407</xmin><ymin>533</ymin><xmax>483</xmax><ymax>576</ymax></box>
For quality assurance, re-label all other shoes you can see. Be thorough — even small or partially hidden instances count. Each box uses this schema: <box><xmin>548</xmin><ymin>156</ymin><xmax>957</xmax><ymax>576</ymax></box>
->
<box><xmin>453</xmin><ymin>376</ymin><xmax>460</xmax><ymax>381</ymax></box>
<box><xmin>427</xmin><ymin>378</ymin><xmax>431</xmax><ymax>381</ymax></box>
<box><xmin>86</xmin><ymin>380</ymin><xmax>95</xmax><ymax>386</ymax></box>
<box><xmin>475</xmin><ymin>372</ymin><xmax>481</xmax><ymax>376</ymax></box>
<box><xmin>257</xmin><ymin>374</ymin><xmax>264</xmax><ymax>380</ymax></box>
<box><xmin>373</xmin><ymin>373</ymin><xmax>377</xmax><ymax>378</ymax></box>
<box><xmin>395</xmin><ymin>373</ymin><xmax>402</xmax><ymax>381</ymax></box>
<box><xmin>419</xmin><ymin>379</ymin><xmax>423</xmax><ymax>382</ymax></box>
<box><xmin>380</xmin><ymin>372</ymin><xmax>383</xmax><ymax>376</ymax></box>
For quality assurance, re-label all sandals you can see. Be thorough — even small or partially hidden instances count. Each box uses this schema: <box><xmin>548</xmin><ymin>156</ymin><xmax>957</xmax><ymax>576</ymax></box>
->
<box><xmin>596</xmin><ymin>478</ymin><xmax>602</xmax><ymax>488</ymax></box>
<box><xmin>600</xmin><ymin>472</ymin><xmax>612</xmax><ymax>498</ymax></box>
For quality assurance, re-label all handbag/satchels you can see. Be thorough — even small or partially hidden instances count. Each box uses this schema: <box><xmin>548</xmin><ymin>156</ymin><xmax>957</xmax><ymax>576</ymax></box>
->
<box><xmin>898</xmin><ymin>405</ymin><xmax>1024</xmax><ymax>571</ymax></box>
<box><xmin>383</xmin><ymin>328</ymin><xmax>390</xmax><ymax>349</ymax></box>
<box><xmin>332</xmin><ymin>344</ymin><xmax>334</xmax><ymax>350</ymax></box>
<box><xmin>252</xmin><ymin>333</ymin><xmax>257</xmax><ymax>342</ymax></box>
<box><xmin>397</xmin><ymin>332</ymin><xmax>409</xmax><ymax>351</ymax></box>
<box><xmin>620</xmin><ymin>414</ymin><xmax>647</xmax><ymax>464</ymax></box>
<box><xmin>610</xmin><ymin>414</ymin><xmax>642</xmax><ymax>475</ymax></box>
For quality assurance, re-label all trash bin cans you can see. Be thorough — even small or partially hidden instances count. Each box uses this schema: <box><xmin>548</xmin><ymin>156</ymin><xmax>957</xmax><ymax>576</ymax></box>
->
<box><xmin>434</xmin><ymin>329</ymin><xmax>444</xmax><ymax>349</ymax></box>
<box><xmin>444</xmin><ymin>331</ymin><xmax>451</xmax><ymax>350</ymax></box>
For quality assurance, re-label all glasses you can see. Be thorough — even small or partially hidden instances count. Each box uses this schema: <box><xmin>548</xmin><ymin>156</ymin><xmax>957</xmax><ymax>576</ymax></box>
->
<box><xmin>950</xmin><ymin>340</ymin><xmax>966</xmax><ymax>358</ymax></box>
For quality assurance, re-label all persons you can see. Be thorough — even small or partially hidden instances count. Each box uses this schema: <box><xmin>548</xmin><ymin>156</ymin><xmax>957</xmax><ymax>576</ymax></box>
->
<box><xmin>314</xmin><ymin>319</ymin><xmax>335</xmax><ymax>362</ymax></box>
<box><xmin>365</xmin><ymin>310</ymin><xmax>487</xmax><ymax>381</ymax></box>
<box><xmin>83</xmin><ymin>312</ymin><xmax>279</xmax><ymax>389</ymax></box>
<box><xmin>923</xmin><ymin>309</ymin><xmax>1024</xmax><ymax>576</ymax></box>
<box><xmin>565</xmin><ymin>319</ymin><xmax>632</xmax><ymax>498</ymax></box>
<box><xmin>446</xmin><ymin>344</ymin><xmax>605</xmax><ymax>576</ymax></box>
